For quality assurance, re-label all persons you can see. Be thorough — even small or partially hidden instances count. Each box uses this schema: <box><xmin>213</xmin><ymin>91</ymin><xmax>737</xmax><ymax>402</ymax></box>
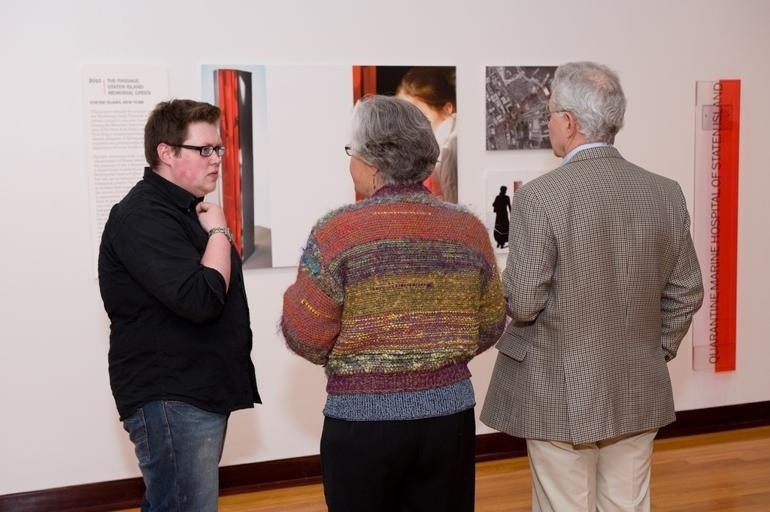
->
<box><xmin>280</xmin><ymin>94</ymin><xmax>508</xmax><ymax>512</ymax></box>
<box><xmin>395</xmin><ymin>66</ymin><xmax>457</xmax><ymax>204</ymax></box>
<box><xmin>479</xmin><ymin>61</ymin><xmax>706</xmax><ymax>512</ymax></box>
<box><xmin>96</xmin><ymin>99</ymin><xmax>262</xmax><ymax>511</ymax></box>
<box><xmin>491</xmin><ymin>186</ymin><xmax>511</xmax><ymax>249</ymax></box>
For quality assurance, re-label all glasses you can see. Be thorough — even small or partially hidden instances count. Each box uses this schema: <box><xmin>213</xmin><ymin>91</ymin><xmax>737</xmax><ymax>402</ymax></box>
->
<box><xmin>342</xmin><ymin>144</ymin><xmax>362</xmax><ymax>158</ymax></box>
<box><xmin>162</xmin><ymin>142</ymin><xmax>226</xmax><ymax>158</ymax></box>
<box><xmin>542</xmin><ymin>107</ymin><xmax>572</xmax><ymax>121</ymax></box>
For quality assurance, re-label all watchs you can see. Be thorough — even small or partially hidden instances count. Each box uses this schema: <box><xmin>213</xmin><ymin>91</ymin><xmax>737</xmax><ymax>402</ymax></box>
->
<box><xmin>207</xmin><ymin>227</ymin><xmax>235</xmax><ymax>245</ymax></box>
